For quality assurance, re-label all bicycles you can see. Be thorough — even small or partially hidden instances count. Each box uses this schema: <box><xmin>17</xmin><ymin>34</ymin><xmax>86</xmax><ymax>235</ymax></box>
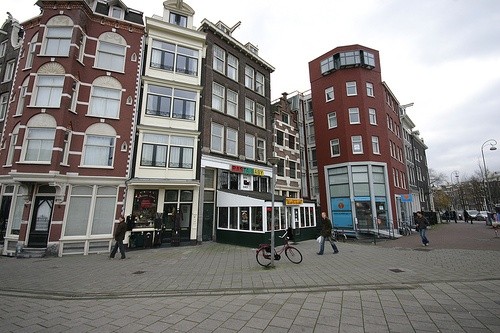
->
<box><xmin>256</xmin><ymin>229</ymin><xmax>303</xmax><ymax>267</ymax></box>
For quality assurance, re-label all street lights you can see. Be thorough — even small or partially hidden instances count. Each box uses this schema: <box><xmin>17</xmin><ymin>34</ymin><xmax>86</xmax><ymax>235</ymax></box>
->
<box><xmin>451</xmin><ymin>170</ymin><xmax>460</xmax><ymax>210</ymax></box>
<box><xmin>266</xmin><ymin>157</ymin><xmax>281</xmax><ymax>269</ymax></box>
<box><xmin>481</xmin><ymin>138</ymin><xmax>498</xmax><ymax>238</ymax></box>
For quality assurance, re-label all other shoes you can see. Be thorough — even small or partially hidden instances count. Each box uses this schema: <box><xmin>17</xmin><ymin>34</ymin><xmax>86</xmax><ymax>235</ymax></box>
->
<box><xmin>426</xmin><ymin>243</ymin><xmax>429</xmax><ymax>246</ymax></box>
<box><xmin>317</xmin><ymin>252</ymin><xmax>323</xmax><ymax>255</ymax></box>
<box><xmin>333</xmin><ymin>250</ymin><xmax>338</xmax><ymax>254</ymax></box>
<box><xmin>423</xmin><ymin>244</ymin><xmax>425</xmax><ymax>246</ymax></box>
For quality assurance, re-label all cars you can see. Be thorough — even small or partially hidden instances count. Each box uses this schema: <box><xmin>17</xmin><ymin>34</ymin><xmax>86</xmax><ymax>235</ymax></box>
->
<box><xmin>442</xmin><ymin>209</ymin><xmax>490</xmax><ymax>221</ymax></box>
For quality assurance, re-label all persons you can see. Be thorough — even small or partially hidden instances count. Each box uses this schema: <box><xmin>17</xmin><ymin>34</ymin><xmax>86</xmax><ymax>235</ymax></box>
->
<box><xmin>415</xmin><ymin>211</ymin><xmax>430</xmax><ymax>246</ymax></box>
<box><xmin>453</xmin><ymin>211</ymin><xmax>457</xmax><ymax>223</ymax></box>
<box><xmin>464</xmin><ymin>210</ymin><xmax>468</xmax><ymax>223</ymax></box>
<box><xmin>110</xmin><ymin>216</ymin><xmax>126</xmax><ymax>259</ymax></box>
<box><xmin>317</xmin><ymin>211</ymin><xmax>338</xmax><ymax>255</ymax></box>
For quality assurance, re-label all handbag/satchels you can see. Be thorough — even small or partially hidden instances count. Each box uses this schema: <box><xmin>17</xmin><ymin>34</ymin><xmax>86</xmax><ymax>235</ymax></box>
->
<box><xmin>316</xmin><ymin>236</ymin><xmax>322</xmax><ymax>243</ymax></box>
